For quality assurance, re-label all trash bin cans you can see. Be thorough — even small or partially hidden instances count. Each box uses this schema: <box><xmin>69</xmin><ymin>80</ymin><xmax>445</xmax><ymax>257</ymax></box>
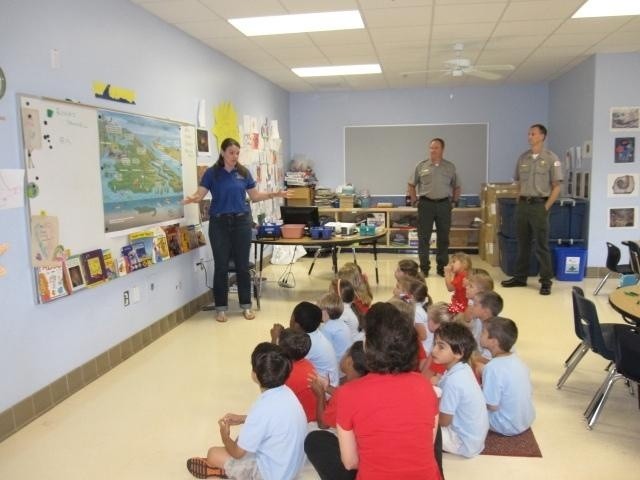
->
<box><xmin>554</xmin><ymin>247</ymin><xmax>587</xmax><ymax>281</ymax></box>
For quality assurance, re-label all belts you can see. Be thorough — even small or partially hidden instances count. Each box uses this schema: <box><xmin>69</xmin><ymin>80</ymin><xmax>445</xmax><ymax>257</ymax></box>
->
<box><xmin>519</xmin><ymin>195</ymin><xmax>548</xmax><ymax>202</ymax></box>
<box><xmin>420</xmin><ymin>196</ymin><xmax>449</xmax><ymax>204</ymax></box>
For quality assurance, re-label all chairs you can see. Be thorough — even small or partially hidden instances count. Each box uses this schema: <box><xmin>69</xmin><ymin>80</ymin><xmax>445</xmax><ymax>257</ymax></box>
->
<box><xmin>556</xmin><ymin>285</ymin><xmax>640</xmax><ymax>428</ymax></box>
<box><xmin>593</xmin><ymin>240</ymin><xmax>634</xmax><ymax>296</ymax></box>
<box><xmin>621</xmin><ymin>240</ymin><xmax>640</xmax><ymax>279</ymax></box>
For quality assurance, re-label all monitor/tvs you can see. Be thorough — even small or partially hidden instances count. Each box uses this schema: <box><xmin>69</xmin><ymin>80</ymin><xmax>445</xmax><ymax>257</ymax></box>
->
<box><xmin>281</xmin><ymin>206</ymin><xmax>320</xmax><ymax>227</ymax></box>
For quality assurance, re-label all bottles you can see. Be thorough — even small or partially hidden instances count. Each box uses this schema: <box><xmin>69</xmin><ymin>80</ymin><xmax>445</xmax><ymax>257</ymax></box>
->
<box><xmin>335</xmin><ymin>198</ymin><xmax>340</xmax><ymax>207</ymax></box>
<box><xmin>368</xmin><ymin>224</ymin><xmax>375</xmax><ymax>236</ymax></box>
<box><xmin>360</xmin><ymin>222</ymin><xmax>365</xmax><ymax>236</ymax></box>
<box><xmin>406</xmin><ymin>191</ymin><xmax>411</xmax><ymax>206</ymax></box>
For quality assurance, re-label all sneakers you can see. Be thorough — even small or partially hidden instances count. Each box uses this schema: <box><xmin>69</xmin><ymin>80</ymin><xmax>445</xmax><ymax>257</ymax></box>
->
<box><xmin>215</xmin><ymin>313</ymin><xmax>228</xmax><ymax>322</ymax></box>
<box><xmin>185</xmin><ymin>456</ymin><xmax>230</xmax><ymax>480</ymax></box>
<box><xmin>501</xmin><ymin>277</ymin><xmax>528</xmax><ymax>287</ymax></box>
<box><xmin>539</xmin><ymin>284</ymin><xmax>552</xmax><ymax>295</ymax></box>
<box><xmin>420</xmin><ymin>270</ymin><xmax>429</xmax><ymax>278</ymax></box>
<box><xmin>242</xmin><ymin>310</ymin><xmax>256</xmax><ymax>320</ymax></box>
<box><xmin>436</xmin><ymin>264</ymin><xmax>445</xmax><ymax>277</ymax></box>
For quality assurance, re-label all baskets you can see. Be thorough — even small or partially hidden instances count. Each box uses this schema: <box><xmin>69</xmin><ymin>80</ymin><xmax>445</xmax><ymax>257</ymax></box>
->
<box><xmin>256</xmin><ymin>222</ymin><xmax>283</xmax><ymax>239</ymax></box>
<box><xmin>279</xmin><ymin>223</ymin><xmax>306</xmax><ymax>239</ymax></box>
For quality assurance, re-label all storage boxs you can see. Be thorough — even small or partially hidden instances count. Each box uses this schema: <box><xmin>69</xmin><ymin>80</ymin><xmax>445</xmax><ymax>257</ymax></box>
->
<box><xmin>479</xmin><ymin>183</ymin><xmax>588</xmax><ymax>281</ymax></box>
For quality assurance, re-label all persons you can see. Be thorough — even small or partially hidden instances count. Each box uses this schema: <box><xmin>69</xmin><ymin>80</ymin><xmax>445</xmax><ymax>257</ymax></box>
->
<box><xmin>409</xmin><ymin>138</ymin><xmax>461</xmax><ymax>278</ymax></box>
<box><xmin>180</xmin><ymin>138</ymin><xmax>295</xmax><ymax>322</ymax></box>
<box><xmin>501</xmin><ymin>124</ymin><xmax>563</xmax><ymax>295</ymax></box>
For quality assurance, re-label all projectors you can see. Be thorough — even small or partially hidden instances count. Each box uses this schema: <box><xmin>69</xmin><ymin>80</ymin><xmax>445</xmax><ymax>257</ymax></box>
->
<box><xmin>325</xmin><ymin>221</ymin><xmax>357</xmax><ymax>235</ymax></box>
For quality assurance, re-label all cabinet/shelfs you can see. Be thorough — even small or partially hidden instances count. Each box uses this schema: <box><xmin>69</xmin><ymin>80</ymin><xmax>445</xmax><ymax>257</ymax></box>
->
<box><xmin>283</xmin><ymin>206</ymin><xmax>479</xmax><ymax>249</ymax></box>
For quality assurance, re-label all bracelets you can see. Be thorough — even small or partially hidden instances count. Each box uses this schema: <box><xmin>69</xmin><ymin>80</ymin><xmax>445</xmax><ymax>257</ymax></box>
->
<box><xmin>413</xmin><ymin>200</ymin><xmax>419</xmax><ymax>206</ymax></box>
<box><xmin>451</xmin><ymin>200</ymin><xmax>458</xmax><ymax>207</ymax></box>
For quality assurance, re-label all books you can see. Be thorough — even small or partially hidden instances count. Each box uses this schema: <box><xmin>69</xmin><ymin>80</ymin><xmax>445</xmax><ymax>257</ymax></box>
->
<box><xmin>284</xmin><ymin>170</ymin><xmax>356</xmax><ymax>209</ymax></box>
<box><xmin>389</xmin><ymin>215</ymin><xmax>420</xmax><ymax>247</ymax></box>
<box><xmin>33</xmin><ymin>222</ymin><xmax>206</xmax><ymax>305</ymax></box>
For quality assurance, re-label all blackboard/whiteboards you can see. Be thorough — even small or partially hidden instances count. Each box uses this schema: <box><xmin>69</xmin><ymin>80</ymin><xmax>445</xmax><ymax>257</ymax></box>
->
<box><xmin>16</xmin><ymin>92</ymin><xmax>202</xmax><ymax>305</ymax></box>
<box><xmin>343</xmin><ymin>122</ymin><xmax>490</xmax><ymax>197</ymax></box>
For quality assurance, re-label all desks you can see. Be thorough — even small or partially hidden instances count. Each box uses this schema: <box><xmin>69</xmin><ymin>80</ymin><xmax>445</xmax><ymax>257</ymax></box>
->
<box><xmin>251</xmin><ymin>225</ymin><xmax>388</xmax><ymax>311</ymax></box>
